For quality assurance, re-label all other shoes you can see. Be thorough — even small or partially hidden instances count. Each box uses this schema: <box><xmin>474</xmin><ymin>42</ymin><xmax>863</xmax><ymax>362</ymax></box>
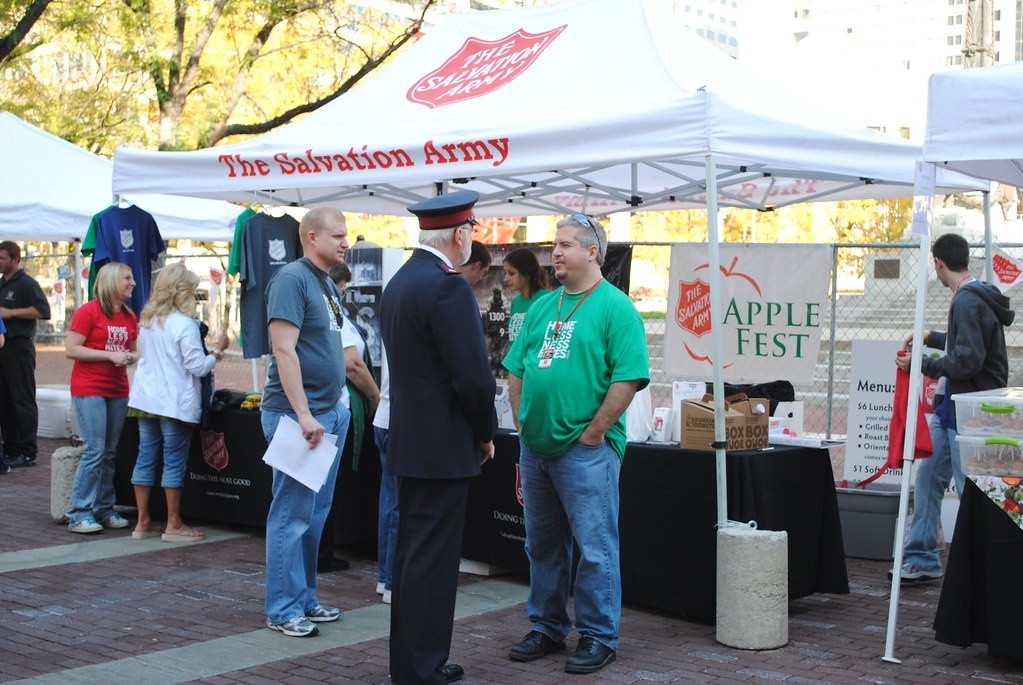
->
<box><xmin>316</xmin><ymin>557</ymin><xmax>350</xmax><ymax>575</ymax></box>
<box><xmin>441</xmin><ymin>663</ymin><xmax>464</xmax><ymax>682</ymax></box>
<box><xmin>6</xmin><ymin>453</ymin><xmax>38</xmax><ymax>469</ymax></box>
<box><xmin>381</xmin><ymin>587</ymin><xmax>392</xmax><ymax>604</ymax></box>
<box><xmin>375</xmin><ymin>582</ymin><xmax>386</xmax><ymax>594</ymax></box>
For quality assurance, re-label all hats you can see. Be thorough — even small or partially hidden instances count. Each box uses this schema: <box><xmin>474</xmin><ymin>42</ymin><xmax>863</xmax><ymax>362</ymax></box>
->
<box><xmin>406</xmin><ymin>188</ymin><xmax>484</xmax><ymax>230</ymax></box>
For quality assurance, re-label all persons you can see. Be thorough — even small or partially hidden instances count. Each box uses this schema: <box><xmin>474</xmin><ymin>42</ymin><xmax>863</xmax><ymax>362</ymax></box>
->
<box><xmin>381</xmin><ymin>190</ymin><xmax>498</xmax><ymax>685</ymax></box>
<box><xmin>502</xmin><ymin>249</ymin><xmax>553</xmax><ymax>379</ymax></box>
<box><xmin>128</xmin><ymin>262</ymin><xmax>229</xmax><ymax>541</ymax></box>
<box><xmin>0</xmin><ymin>241</ymin><xmax>51</xmax><ymax>473</ymax></box>
<box><xmin>887</xmin><ymin>234</ymin><xmax>1014</xmax><ymax>581</ymax></box>
<box><xmin>317</xmin><ymin>264</ymin><xmax>378</xmax><ymax>573</ymax></box>
<box><xmin>499</xmin><ymin>215</ymin><xmax>650</xmax><ymax>681</ymax></box>
<box><xmin>65</xmin><ymin>262</ymin><xmax>139</xmax><ymax>530</ymax></box>
<box><xmin>259</xmin><ymin>205</ymin><xmax>350</xmax><ymax>637</ymax></box>
<box><xmin>373</xmin><ymin>240</ymin><xmax>491</xmax><ymax>604</ymax></box>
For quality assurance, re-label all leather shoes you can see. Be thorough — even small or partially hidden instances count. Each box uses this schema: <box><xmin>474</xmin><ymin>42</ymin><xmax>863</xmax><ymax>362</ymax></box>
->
<box><xmin>565</xmin><ymin>636</ymin><xmax>617</xmax><ymax>674</ymax></box>
<box><xmin>509</xmin><ymin>628</ymin><xmax>566</xmax><ymax>661</ymax></box>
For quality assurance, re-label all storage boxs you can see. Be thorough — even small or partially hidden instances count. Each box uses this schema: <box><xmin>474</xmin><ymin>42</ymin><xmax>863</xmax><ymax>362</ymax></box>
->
<box><xmin>679</xmin><ymin>390</ymin><xmax>771</xmax><ymax>453</ymax></box>
<box><xmin>954</xmin><ymin>435</ymin><xmax>1023</xmax><ymax>478</ymax></box>
<box><xmin>949</xmin><ymin>386</ymin><xmax>1023</xmax><ymax>438</ymax></box>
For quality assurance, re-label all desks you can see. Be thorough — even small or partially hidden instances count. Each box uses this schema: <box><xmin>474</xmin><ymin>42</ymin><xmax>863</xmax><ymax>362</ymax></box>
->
<box><xmin>932</xmin><ymin>473</ymin><xmax>1023</xmax><ymax>663</ymax></box>
<box><xmin>121</xmin><ymin>408</ymin><xmax>848</xmax><ymax>629</ymax></box>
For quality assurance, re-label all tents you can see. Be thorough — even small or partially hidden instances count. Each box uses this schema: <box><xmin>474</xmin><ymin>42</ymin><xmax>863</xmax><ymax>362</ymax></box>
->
<box><xmin>882</xmin><ymin>60</ymin><xmax>1023</xmax><ymax>662</ymax></box>
<box><xmin>114</xmin><ymin>0</ymin><xmax>992</xmax><ymax>533</ymax></box>
<box><xmin>0</xmin><ymin>111</ymin><xmax>246</xmax><ymax>308</ymax></box>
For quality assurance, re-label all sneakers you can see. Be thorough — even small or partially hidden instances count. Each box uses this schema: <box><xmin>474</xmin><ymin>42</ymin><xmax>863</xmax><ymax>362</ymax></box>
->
<box><xmin>267</xmin><ymin>612</ymin><xmax>319</xmax><ymax>638</ymax></box>
<box><xmin>888</xmin><ymin>562</ymin><xmax>944</xmax><ymax>581</ymax></box>
<box><xmin>96</xmin><ymin>514</ymin><xmax>129</xmax><ymax>529</ymax></box>
<box><xmin>67</xmin><ymin>518</ymin><xmax>104</xmax><ymax>533</ymax></box>
<box><xmin>305</xmin><ymin>604</ymin><xmax>342</xmax><ymax>621</ymax></box>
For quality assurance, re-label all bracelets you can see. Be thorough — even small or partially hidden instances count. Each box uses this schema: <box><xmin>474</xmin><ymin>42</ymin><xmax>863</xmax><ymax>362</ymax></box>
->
<box><xmin>925</xmin><ymin>332</ymin><xmax>928</xmax><ymax>345</ymax></box>
<box><xmin>210</xmin><ymin>348</ymin><xmax>223</xmax><ymax>359</ymax></box>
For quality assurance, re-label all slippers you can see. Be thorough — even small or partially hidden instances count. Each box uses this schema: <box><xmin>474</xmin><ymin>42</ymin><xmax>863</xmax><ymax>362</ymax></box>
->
<box><xmin>129</xmin><ymin>520</ymin><xmax>163</xmax><ymax>539</ymax></box>
<box><xmin>160</xmin><ymin>525</ymin><xmax>208</xmax><ymax>542</ymax></box>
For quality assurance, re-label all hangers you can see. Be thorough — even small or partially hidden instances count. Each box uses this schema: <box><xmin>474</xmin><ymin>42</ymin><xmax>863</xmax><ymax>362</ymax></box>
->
<box><xmin>250</xmin><ymin>188</ymin><xmax>287</xmax><ymax>216</ymax></box>
<box><xmin>112</xmin><ymin>192</ymin><xmax>133</xmax><ymax>209</ymax></box>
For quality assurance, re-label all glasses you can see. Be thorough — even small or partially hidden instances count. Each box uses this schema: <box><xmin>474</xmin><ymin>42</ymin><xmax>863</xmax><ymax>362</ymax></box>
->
<box><xmin>567</xmin><ymin>212</ymin><xmax>602</xmax><ymax>256</ymax></box>
